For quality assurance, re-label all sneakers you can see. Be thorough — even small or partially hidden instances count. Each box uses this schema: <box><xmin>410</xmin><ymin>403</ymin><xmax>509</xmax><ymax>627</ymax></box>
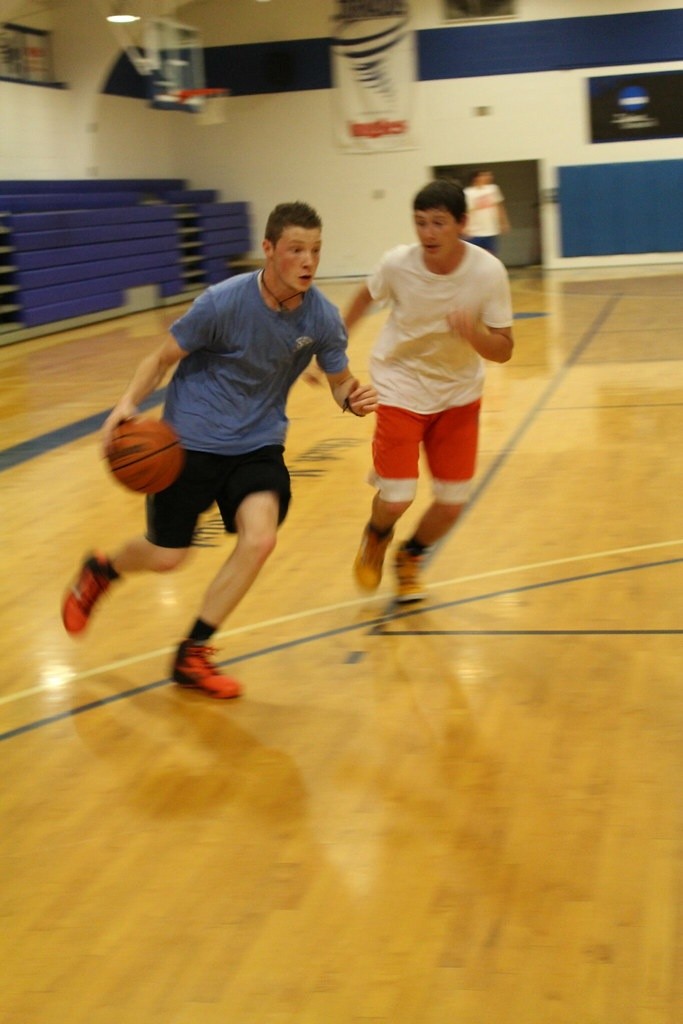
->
<box><xmin>172</xmin><ymin>641</ymin><xmax>242</xmax><ymax>700</ymax></box>
<box><xmin>61</xmin><ymin>548</ymin><xmax>121</xmax><ymax>634</ymax></box>
<box><xmin>395</xmin><ymin>541</ymin><xmax>429</xmax><ymax>602</ymax></box>
<box><xmin>355</xmin><ymin>522</ymin><xmax>395</xmax><ymax>589</ymax></box>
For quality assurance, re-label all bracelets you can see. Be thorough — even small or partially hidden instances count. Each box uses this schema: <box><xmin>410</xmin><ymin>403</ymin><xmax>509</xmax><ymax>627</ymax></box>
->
<box><xmin>342</xmin><ymin>396</ymin><xmax>365</xmax><ymax>417</ymax></box>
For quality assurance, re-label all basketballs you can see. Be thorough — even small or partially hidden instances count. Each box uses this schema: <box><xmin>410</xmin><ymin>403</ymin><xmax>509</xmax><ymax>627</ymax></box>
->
<box><xmin>105</xmin><ymin>415</ymin><xmax>183</xmax><ymax>496</ymax></box>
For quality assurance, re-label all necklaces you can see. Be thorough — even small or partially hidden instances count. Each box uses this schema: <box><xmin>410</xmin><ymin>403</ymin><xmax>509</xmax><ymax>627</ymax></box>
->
<box><xmin>262</xmin><ymin>268</ymin><xmax>304</xmax><ymax>312</ymax></box>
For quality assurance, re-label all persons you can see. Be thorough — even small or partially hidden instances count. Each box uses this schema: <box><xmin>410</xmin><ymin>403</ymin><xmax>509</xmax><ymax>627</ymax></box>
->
<box><xmin>305</xmin><ymin>182</ymin><xmax>516</xmax><ymax>603</ymax></box>
<box><xmin>462</xmin><ymin>169</ymin><xmax>510</xmax><ymax>257</ymax></box>
<box><xmin>61</xmin><ymin>201</ymin><xmax>380</xmax><ymax>699</ymax></box>
